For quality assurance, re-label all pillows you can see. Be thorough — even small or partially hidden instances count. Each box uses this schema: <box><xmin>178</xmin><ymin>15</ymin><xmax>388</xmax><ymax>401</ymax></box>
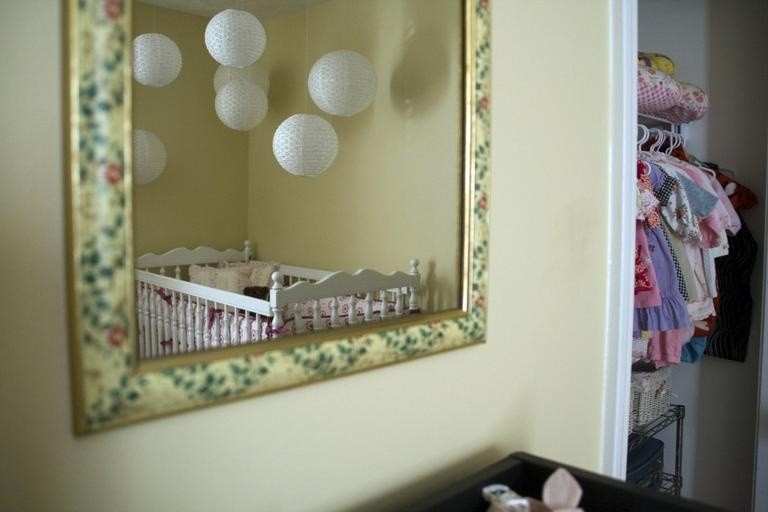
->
<box><xmin>225</xmin><ymin>261</ymin><xmax>281</xmax><ymax>287</ymax></box>
<box><xmin>188</xmin><ymin>265</ymin><xmax>252</xmax><ymax>294</ymax></box>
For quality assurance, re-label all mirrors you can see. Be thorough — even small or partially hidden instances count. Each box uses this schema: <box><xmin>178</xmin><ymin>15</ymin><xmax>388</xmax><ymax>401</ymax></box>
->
<box><xmin>61</xmin><ymin>1</ymin><xmax>490</xmax><ymax>435</ymax></box>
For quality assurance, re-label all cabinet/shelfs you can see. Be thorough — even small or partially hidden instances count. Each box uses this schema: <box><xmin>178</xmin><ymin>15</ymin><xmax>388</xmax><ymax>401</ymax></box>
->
<box><xmin>627</xmin><ymin>406</ymin><xmax>685</xmax><ymax>497</ymax></box>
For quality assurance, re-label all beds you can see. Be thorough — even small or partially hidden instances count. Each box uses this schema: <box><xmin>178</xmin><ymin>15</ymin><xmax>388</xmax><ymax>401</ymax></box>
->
<box><xmin>134</xmin><ymin>239</ymin><xmax>420</xmax><ymax>364</ymax></box>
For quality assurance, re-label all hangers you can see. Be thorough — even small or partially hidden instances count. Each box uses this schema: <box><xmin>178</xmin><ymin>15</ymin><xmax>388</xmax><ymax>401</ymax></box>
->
<box><xmin>636</xmin><ymin>123</ymin><xmax>736</xmax><ymax>182</ymax></box>
